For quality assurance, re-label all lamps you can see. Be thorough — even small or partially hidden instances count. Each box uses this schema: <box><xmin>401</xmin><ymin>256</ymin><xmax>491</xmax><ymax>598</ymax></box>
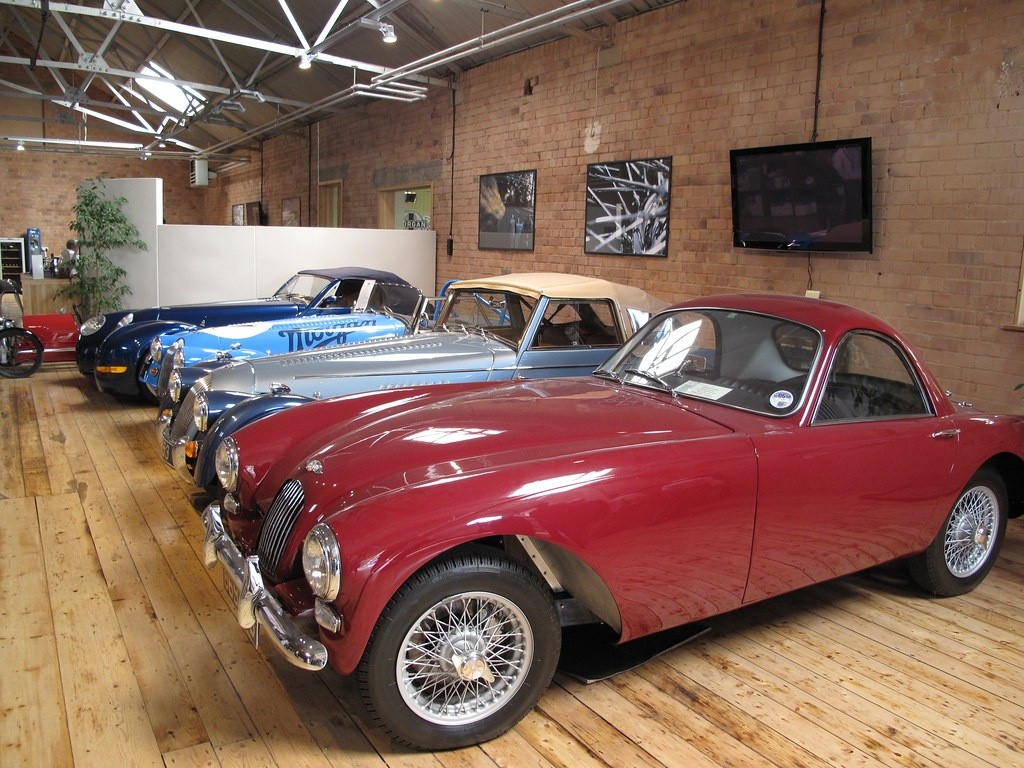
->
<box><xmin>17</xmin><ymin>141</ymin><xmax>26</xmax><ymax>150</ymax></box>
<box><xmin>298</xmin><ymin>57</ymin><xmax>314</xmax><ymax>70</ymax></box>
<box><xmin>359</xmin><ymin>18</ymin><xmax>398</xmax><ymax>44</ymax></box>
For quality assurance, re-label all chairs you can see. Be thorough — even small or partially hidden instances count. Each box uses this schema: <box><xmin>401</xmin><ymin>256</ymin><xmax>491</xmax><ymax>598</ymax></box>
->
<box><xmin>536</xmin><ymin>326</ymin><xmax>572</xmax><ymax>346</ymax></box>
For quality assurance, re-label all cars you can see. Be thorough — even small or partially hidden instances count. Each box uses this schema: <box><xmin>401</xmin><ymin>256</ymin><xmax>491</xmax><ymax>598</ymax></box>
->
<box><xmin>157</xmin><ymin>270</ymin><xmax>715</xmax><ymax>513</ymax></box>
<box><xmin>202</xmin><ymin>293</ymin><xmax>1024</xmax><ymax>754</ymax></box>
<box><xmin>138</xmin><ymin>280</ymin><xmax>517</xmax><ymax>399</ymax></box>
<box><xmin>74</xmin><ymin>266</ymin><xmax>436</xmax><ymax>407</ymax></box>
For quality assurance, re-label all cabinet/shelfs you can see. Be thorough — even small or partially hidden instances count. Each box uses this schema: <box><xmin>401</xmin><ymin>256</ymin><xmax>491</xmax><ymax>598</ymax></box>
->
<box><xmin>0</xmin><ymin>237</ymin><xmax>27</xmax><ymax>280</ymax></box>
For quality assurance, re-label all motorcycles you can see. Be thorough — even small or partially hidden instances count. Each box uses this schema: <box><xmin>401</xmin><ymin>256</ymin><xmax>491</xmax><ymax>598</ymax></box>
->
<box><xmin>0</xmin><ymin>279</ymin><xmax>45</xmax><ymax>379</ymax></box>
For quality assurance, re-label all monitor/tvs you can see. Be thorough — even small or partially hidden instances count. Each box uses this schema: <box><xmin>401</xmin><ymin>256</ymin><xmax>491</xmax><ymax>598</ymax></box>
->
<box><xmin>246</xmin><ymin>201</ymin><xmax>264</xmax><ymax>225</ymax></box>
<box><xmin>730</xmin><ymin>136</ymin><xmax>874</xmax><ymax>252</ymax></box>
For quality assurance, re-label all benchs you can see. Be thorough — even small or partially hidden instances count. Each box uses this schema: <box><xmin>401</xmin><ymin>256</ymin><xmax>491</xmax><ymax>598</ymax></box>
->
<box><xmin>716</xmin><ymin>376</ymin><xmax>902</xmax><ymax>421</ymax></box>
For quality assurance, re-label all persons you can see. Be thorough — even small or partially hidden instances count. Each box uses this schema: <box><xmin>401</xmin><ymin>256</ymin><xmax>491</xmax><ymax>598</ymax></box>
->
<box><xmin>59</xmin><ymin>238</ymin><xmax>80</xmax><ymax>275</ymax></box>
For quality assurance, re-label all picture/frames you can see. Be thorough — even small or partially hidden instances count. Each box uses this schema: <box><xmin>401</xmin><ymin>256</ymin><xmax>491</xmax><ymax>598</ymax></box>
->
<box><xmin>584</xmin><ymin>156</ymin><xmax>673</xmax><ymax>259</ymax></box>
<box><xmin>281</xmin><ymin>197</ymin><xmax>302</xmax><ymax>227</ymax></box>
<box><xmin>232</xmin><ymin>204</ymin><xmax>245</xmax><ymax>226</ymax></box>
<box><xmin>478</xmin><ymin>168</ymin><xmax>537</xmax><ymax>252</ymax></box>
